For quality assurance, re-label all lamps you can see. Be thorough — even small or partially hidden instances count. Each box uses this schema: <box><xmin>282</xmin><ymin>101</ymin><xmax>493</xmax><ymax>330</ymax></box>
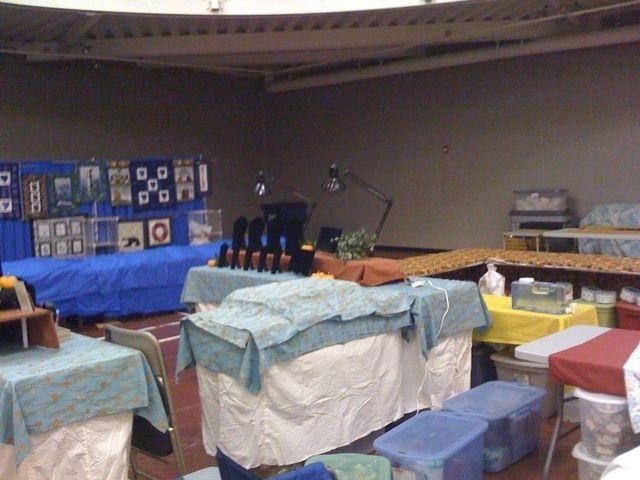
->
<box><xmin>250</xmin><ymin>169</ymin><xmax>319</xmax><ymax>231</ymax></box>
<box><xmin>321</xmin><ymin>162</ymin><xmax>392</xmax><ymax>254</ymax></box>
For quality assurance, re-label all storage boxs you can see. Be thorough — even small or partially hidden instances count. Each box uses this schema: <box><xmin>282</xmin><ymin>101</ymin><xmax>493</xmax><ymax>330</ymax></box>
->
<box><xmin>509</xmin><ymin>210</ymin><xmax>575</xmax><ymax>233</ymax></box>
<box><xmin>513</xmin><ymin>188</ymin><xmax>570</xmax><ymax>210</ymax></box>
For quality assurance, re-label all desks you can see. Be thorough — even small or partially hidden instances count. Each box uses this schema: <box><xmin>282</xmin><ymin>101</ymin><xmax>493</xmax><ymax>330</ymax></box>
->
<box><xmin>473</xmin><ymin>295</ymin><xmax>599</xmax><ymax>344</ymax></box>
<box><xmin>502</xmin><ymin>231</ymin><xmax>542</xmax><ymax>252</ymax></box>
<box><xmin>397</xmin><ymin>245</ymin><xmax>640</xmax><ymax>287</ymax></box>
<box><xmin>176</xmin><ymin>276</ymin><xmax>492</xmax><ymax>469</ymax></box>
<box><xmin>544</xmin><ymin>227</ymin><xmax>640</xmax><ymax>257</ymax></box>
<box><xmin>0</xmin><ymin>331</ymin><xmax>169</xmax><ymax>480</ymax></box>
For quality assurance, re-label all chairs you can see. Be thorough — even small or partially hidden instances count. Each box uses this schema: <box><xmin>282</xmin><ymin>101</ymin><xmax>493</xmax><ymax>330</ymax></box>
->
<box><xmin>102</xmin><ymin>317</ymin><xmax>186</xmax><ymax>480</ymax></box>
